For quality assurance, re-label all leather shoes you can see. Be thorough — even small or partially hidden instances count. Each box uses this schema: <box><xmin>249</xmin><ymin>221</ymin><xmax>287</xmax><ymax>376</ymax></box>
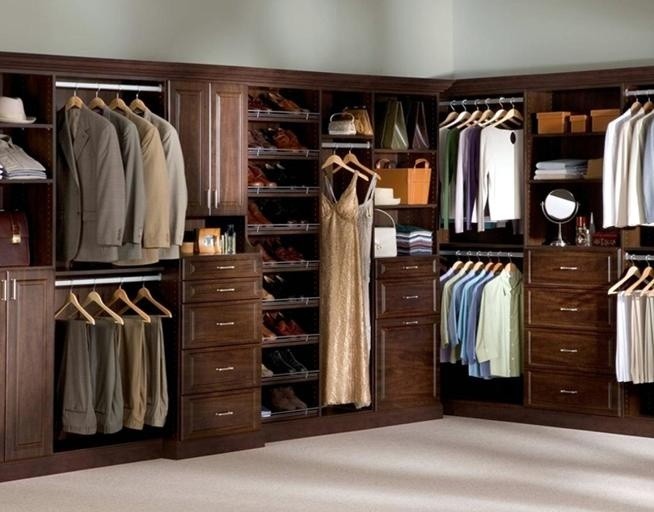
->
<box><xmin>263</xmin><ymin>311</ymin><xmax>318</xmax><ymax>338</ymax></box>
<box><xmin>248</xmin><ymin>92</ymin><xmax>309</xmax><ymax>114</ymax></box>
<box><xmin>247</xmin><ymin>162</ymin><xmax>307</xmax><ymax>189</ymax></box>
<box><xmin>247</xmin><ymin>125</ymin><xmax>306</xmax><ymax>153</ymax></box>
<box><xmin>263</xmin><ymin>274</ymin><xmax>318</xmax><ymax>299</ymax></box>
<box><xmin>265</xmin><ymin>387</ymin><xmax>309</xmax><ymax>412</ymax></box>
<box><xmin>261</xmin><ymin>349</ymin><xmax>316</xmax><ymax>377</ymax></box>
<box><xmin>248</xmin><ymin>202</ymin><xmax>317</xmax><ymax>226</ymax></box>
<box><xmin>250</xmin><ymin>237</ymin><xmax>306</xmax><ymax>263</ymax></box>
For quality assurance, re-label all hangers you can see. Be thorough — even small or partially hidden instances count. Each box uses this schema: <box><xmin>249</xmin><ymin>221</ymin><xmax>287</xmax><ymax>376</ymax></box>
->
<box><xmin>625</xmin><ymin>255</ymin><xmax>653</xmax><ymax>296</ymax></box>
<box><xmin>92</xmin><ymin>286</ymin><xmax>150</xmax><ymax>324</ymax></box>
<box><xmin>116</xmin><ymin>289</ymin><xmax>174</xmax><ymax>320</ymax></box>
<box><xmin>89</xmin><ymin>83</ymin><xmax>103</xmax><ymax>111</ymax></box>
<box><xmin>330</xmin><ymin>144</ymin><xmax>381</xmax><ymax>182</ymax></box>
<box><xmin>108</xmin><ymin>84</ymin><xmax>130</xmax><ymax>115</ymax></box>
<box><xmin>55</xmin><ymin>283</ymin><xmax>98</xmax><ymax>326</ymax></box>
<box><xmin>485</xmin><ymin>98</ymin><xmax>523</xmax><ymax>128</ymax></box>
<box><xmin>639</xmin><ymin>91</ymin><xmax>653</xmax><ymax>114</ymax></box>
<box><xmin>65</xmin><ymin>82</ymin><xmax>83</xmax><ymax>111</ymax></box>
<box><xmin>607</xmin><ymin>255</ymin><xmax>654</xmax><ymax>294</ymax></box>
<box><xmin>626</xmin><ymin>89</ymin><xmax>643</xmax><ymax>114</ymax></box>
<box><xmin>467</xmin><ymin>98</ymin><xmax>510</xmax><ymax>128</ymax></box>
<box><xmin>127</xmin><ymin>86</ymin><xmax>145</xmax><ymax>114</ymax></box>
<box><xmin>71</xmin><ymin>283</ymin><xmax>125</xmax><ymax>324</ymax></box>
<box><xmin>640</xmin><ymin>279</ymin><xmax>653</xmax><ymax>296</ymax></box>
<box><xmin>322</xmin><ymin>142</ymin><xmax>368</xmax><ymax>182</ymax></box>
<box><xmin>480</xmin><ymin>97</ymin><xmax>522</xmax><ymax>128</ymax></box>
<box><xmin>459</xmin><ymin>99</ymin><xmax>482</xmax><ymax>129</ymax></box>
<box><xmin>441</xmin><ymin>101</ymin><xmax>469</xmax><ymax>130</ymax></box>
<box><xmin>435</xmin><ymin>250</ymin><xmax>520</xmax><ymax>287</ymax></box>
<box><xmin>439</xmin><ymin>99</ymin><xmax>462</xmax><ymax>127</ymax></box>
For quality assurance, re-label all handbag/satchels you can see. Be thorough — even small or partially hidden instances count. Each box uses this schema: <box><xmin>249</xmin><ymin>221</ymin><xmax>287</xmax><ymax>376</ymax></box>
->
<box><xmin>328</xmin><ymin>111</ymin><xmax>357</xmax><ymax>135</ymax></box>
<box><xmin>342</xmin><ymin>105</ymin><xmax>375</xmax><ymax>137</ymax></box>
<box><xmin>377</xmin><ymin>97</ymin><xmax>411</xmax><ymax>150</ymax></box>
<box><xmin>374</xmin><ymin>205</ymin><xmax>399</xmax><ymax>259</ymax></box>
<box><xmin>0</xmin><ymin>199</ymin><xmax>32</xmax><ymax>268</ymax></box>
<box><xmin>375</xmin><ymin>157</ymin><xmax>431</xmax><ymax>204</ymax></box>
<box><xmin>405</xmin><ymin>100</ymin><xmax>432</xmax><ymax>150</ymax></box>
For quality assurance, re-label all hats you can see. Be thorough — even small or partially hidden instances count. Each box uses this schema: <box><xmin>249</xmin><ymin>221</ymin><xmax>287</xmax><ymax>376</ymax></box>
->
<box><xmin>0</xmin><ymin>94</ymin><xmax>38</xmax><ymax>125</ymax></box>
<box><xmin>374</xmin><ymin>186</ymin><xmax>402</xmax><ymax>205</ymax></box>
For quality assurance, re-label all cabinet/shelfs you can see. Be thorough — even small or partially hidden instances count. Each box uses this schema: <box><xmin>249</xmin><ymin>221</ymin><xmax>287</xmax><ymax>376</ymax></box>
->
<box><xmin>524</xmin><ymin>249</ymin><xmax>640</xmax><ymax>437</ymax></box>
<box><xmin>171</xmin><ymin>62</ymin><xmax>247</xmax><ymax>216</ymax></box>
<box><xmin>377</xmin><ymin>260</ymin><xmax>442</xmax><ymax>429</ymax></box>
<box><xmin>177</xmin><ymin>250</ymin><xmax>267</xmax><ymax>462</ymax></box>
<box><xmin>324</xmin><ymin>73</ymin><xmax>438</xmax><ymax>263</ymax></box>
<box><xmin>529</xmin><ymin>67</ymin><xmax>637</xmax><ymax>247</ymax></box>
<box><xmin>0</xmin><ymin>51</ymin><xmax>57</xmax><ymax>483</ymax></box>
<box><xmin>244</xmin><ymin>67</ymin><xmax>327</xmax><ymax>443</ymax></box>
